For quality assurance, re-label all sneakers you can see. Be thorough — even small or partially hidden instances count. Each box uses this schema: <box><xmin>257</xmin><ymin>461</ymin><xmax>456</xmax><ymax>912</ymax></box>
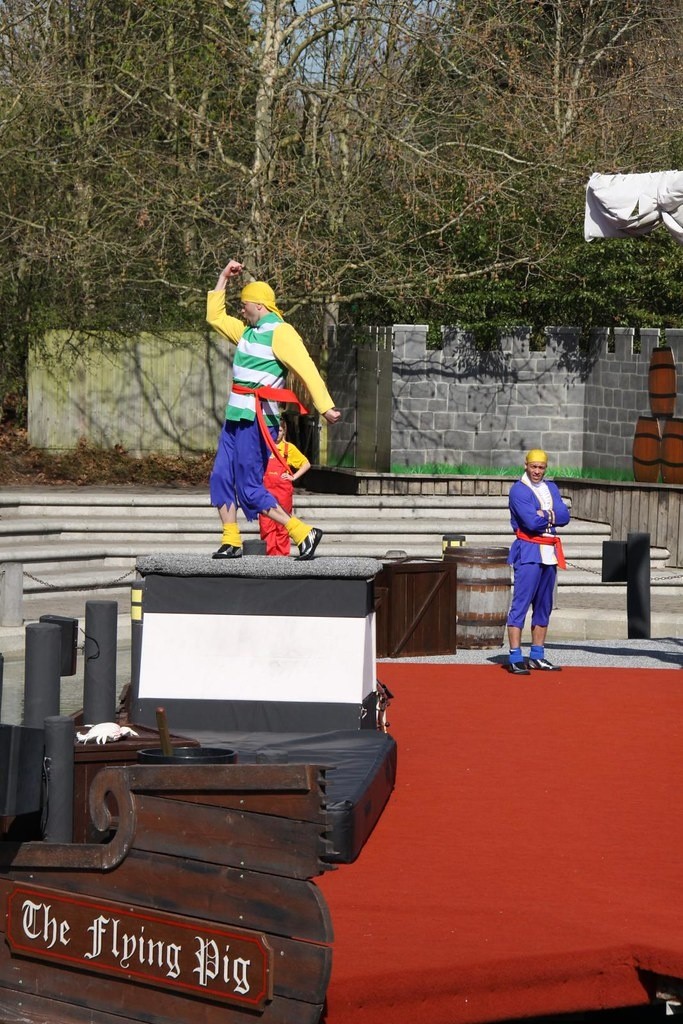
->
<box><xmin>295</xmin><ymin>528</ymin><xmax>323</xmax><ymax>560</ymax></box>
<box><xmin>528</xmin><ymin>658</ymin><xmax>560</xmax><ymax>670</ymax></box>
<box><xmin>509</xmin><ymin>662</ymin><xmax>530</xmax><ymax>674</ymax></box>
<box><xmin>212</xmin><ymin>544</ymin><xmax>242</xmax><ymax>559</ymax></box>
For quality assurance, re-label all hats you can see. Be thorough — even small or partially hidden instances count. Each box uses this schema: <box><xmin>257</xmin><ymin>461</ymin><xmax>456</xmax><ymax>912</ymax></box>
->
<box><xmin>241</xmin><ymin>281</ymin><xmax>283</xmax><ymax>320</ymax></box>
<box><xmin>526</xmin><ymin>449</ymin><xmax>548</xmax><ymax>464</ymax></box>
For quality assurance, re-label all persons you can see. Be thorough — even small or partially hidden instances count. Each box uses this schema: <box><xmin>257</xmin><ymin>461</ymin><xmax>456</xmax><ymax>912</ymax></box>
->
<box><xmin>206</xmin><ymin>259</ymin><xmax>341</xmax><ymax>562</ymax></box>
<box><xmin>508</xmin><ymin>449</ymin><xmax>571</xmax><ymax>675</ymax></box>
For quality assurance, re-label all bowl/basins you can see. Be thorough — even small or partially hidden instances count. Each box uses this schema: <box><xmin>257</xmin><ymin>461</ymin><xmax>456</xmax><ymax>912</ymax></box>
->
<box><xmin>137</xmin><ymin>746</ymin><xmax>237</xmax><ymax>766</ymax></box>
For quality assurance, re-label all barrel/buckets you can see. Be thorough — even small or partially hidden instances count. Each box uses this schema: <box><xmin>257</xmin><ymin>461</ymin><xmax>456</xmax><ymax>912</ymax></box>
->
<box><xmin>445</xmin><ymin>548</ymin><xmax>511</xmax><ymax>650</ymax></box>
